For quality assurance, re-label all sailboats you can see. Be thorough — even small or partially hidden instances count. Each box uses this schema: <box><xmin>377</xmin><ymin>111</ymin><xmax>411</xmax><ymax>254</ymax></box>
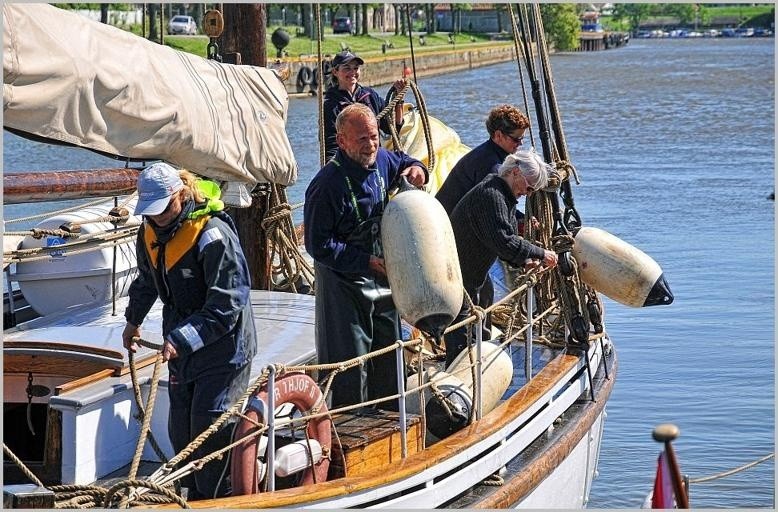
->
<box><xmin>0</xmin><ymin>0</ymin><xmax>616</xmax><ymax>510</ymax></box>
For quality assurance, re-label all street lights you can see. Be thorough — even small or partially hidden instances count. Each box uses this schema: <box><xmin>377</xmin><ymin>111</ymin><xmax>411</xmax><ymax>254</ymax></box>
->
<box><xmin>281</xmin><ymin>7</ymin><xmax>286</xmax><ymax>27</ymax></box>
<box><xmin>324</xmin><ymin>10</ymin><xmax>328</xmax><ymax>25</ymax></box>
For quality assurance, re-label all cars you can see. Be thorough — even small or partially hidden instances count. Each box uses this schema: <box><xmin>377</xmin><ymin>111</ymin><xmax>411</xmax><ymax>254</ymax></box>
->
<box><xmin>167</xmin><ymin>14</ymin><xmax>196</xmax><ymax>35</ymax></box>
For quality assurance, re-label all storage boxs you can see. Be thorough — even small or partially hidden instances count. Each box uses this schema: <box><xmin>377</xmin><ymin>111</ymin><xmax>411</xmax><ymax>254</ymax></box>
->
<box><xmin>263</xmin><ymin>404</ymin><xmax>424</xmax><ymax>477</ymax></box>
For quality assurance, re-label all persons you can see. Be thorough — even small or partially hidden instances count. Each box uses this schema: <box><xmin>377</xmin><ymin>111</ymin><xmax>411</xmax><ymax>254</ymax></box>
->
<box><xmin>435</xmin><ymin>105</ymin><xmax>541</xmax><ymax>341</ymax></box>
<box><xmin>319</xmin><ymin>51</ymin><xmax>407</xmax><ymax>164</ymax></box>
<box><xmin>303</xmin><ymin>103</ymin><xmax>429</xmax><ymax>411</ymax></box>
<box><xmin>122</xmin><ymin>162</ymin><xmax>258</xmax><ymax>501</ymax></box>
<box><xmin>443</xmin><ymin>150</ymin><xmax>550</xmax><ymax>372</ymax></box>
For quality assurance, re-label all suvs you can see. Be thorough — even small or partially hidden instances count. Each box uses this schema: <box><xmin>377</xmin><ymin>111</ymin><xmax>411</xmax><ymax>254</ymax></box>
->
<box><xmin>331</xmin><ymin>16</ymin><xmax>352</xmax><ymax>35</ymax></box>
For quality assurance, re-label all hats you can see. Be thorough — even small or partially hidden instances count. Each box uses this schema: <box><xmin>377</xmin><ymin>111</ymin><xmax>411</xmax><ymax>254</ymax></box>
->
<box><xmin>333</xmin><ymin>51</ymin><xmax>363</xmax><ymax>67</ymax></box>
<box><xmin>134</xmin><ymin>163</ymin><xmax>182</xmax><ymax>216</ymax></box>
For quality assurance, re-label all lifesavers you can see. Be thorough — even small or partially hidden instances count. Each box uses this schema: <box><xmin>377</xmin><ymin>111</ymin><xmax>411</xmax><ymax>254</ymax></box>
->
<box><xmin>604</xmin><ymin>33</ymin><xmax>623</xmax><ymax>44</ymax></box>
<box><xmin>230</xmin><ymin>372</ymin><xmax>331</xmax><ymax>496</ymax></box>
<box><xmin>301</xmin><ymin>60</ymin><xmax>336</xmax><ymax>84</ymax></box>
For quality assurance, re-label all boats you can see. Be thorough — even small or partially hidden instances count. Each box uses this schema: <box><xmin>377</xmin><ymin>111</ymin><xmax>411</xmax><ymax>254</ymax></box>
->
<box><xmin>637</xmin><ymin>28</ymin><xmax>771</xmax><ymax>38</ymax></box>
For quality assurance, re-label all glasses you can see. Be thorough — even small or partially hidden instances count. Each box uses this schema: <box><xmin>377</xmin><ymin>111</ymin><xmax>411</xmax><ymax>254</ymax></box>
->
<box><xmin>519</xmin><ymin>173</ymin><xmax>535</xmax><ymax>192</ymax></box>
<box><xmin>507</xmin><ymin>135</ymin><xmax>523</xmax><ymax>142</ymax></box>
